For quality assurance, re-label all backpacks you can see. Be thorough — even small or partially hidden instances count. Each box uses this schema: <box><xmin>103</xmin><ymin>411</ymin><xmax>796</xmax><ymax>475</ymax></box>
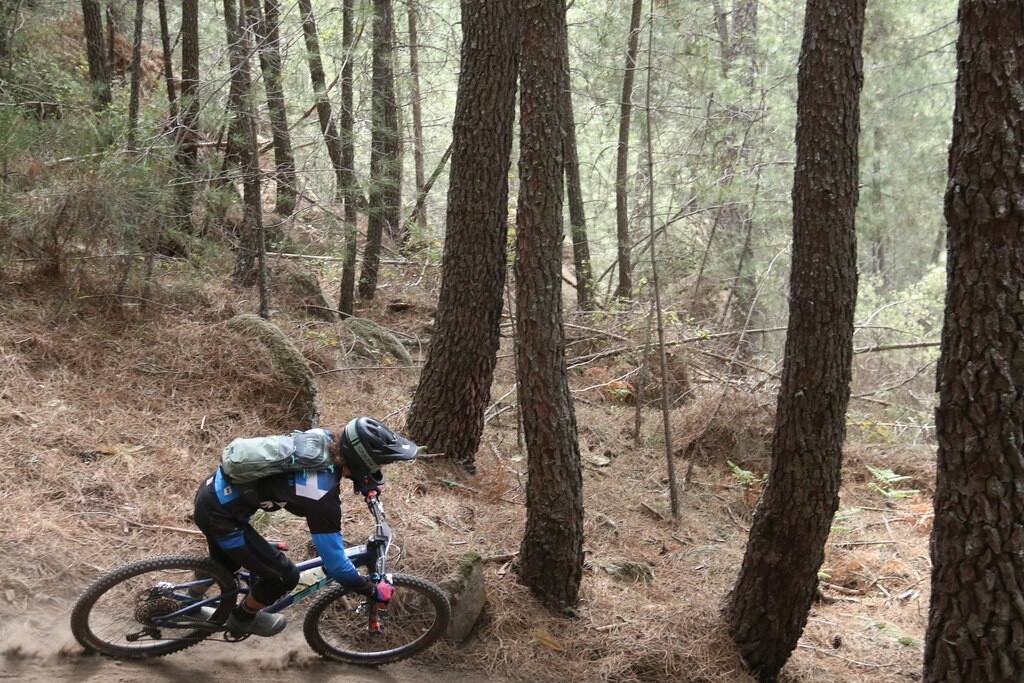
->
<box><xmin>219</xmin><ymin>429</ymin><xmax>342</xmax><ymax>487</ymax></box>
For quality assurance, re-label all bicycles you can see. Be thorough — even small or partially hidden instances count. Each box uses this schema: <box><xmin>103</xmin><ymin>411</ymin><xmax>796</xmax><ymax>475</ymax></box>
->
<box><xmin>70</xmin><ymin>478</ymin><xmax>453</xmax><ymax>667</ymax></box>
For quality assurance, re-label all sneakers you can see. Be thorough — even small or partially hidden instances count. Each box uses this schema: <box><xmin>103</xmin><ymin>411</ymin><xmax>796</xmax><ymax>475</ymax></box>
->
<box><xmin>226</xmin><ymin>610</ymin><xmax>288</xmax><ymax>637</ymax></box>
<box><xmin>186</xmin><ymin>573</ymin><xmax>208</xmax><ymax>599</ymax></box>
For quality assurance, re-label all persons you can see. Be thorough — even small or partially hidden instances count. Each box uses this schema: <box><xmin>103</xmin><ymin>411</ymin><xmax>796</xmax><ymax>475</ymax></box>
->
<box><xmin>179</xmin><ymin>417</ymin><xmax>419</xmax><ymax>636</ymax></box>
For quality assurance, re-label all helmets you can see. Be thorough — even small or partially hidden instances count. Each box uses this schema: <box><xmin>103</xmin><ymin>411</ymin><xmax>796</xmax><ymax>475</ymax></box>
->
<box><xmin>340</xmin><ymin>416</ymin><xmax>417</xmax><ymax>495</ymax></box>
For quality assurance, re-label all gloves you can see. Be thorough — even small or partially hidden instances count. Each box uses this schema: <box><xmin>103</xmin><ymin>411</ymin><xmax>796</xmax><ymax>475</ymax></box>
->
<box><xmin>374</xmin><ymin>579</ymin><xmax>394</xmax><ymax>609</ymax></box>
<box><xmin>359</xmin><ymin>480</ymin><xmax>381</xmax><ymax>501</ymax></box>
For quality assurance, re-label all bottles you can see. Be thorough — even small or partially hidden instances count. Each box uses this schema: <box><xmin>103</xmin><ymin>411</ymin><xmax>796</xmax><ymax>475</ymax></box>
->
<box><xmin>299</xmin><ymin>566</ymin><xmax>328</xmax><ymax>585</ymax></box>
